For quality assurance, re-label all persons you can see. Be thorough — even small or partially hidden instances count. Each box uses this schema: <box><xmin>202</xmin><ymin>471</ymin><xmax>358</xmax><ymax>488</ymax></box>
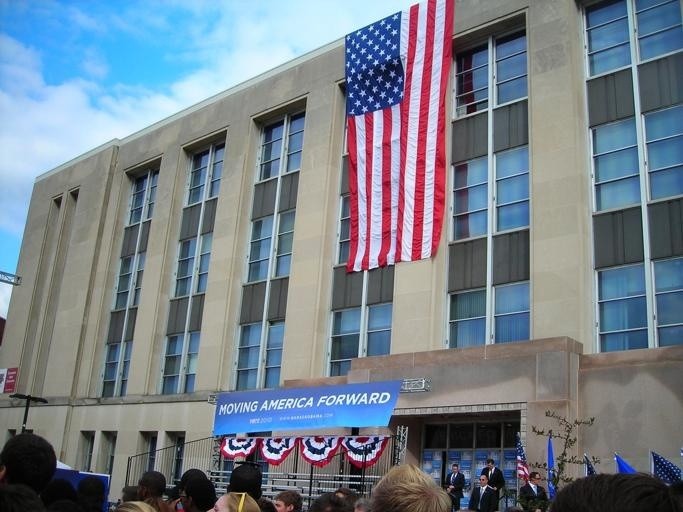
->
<box><xmin>550</xmin><ymin>471</ymin><xmax>674</xmax><ymax>512</ymax></box>
<box><xmin>0</xmin><ymin>432</ymin><xmax>106</xmax><ymax>511</ymax></box>
<box><xmin>116</xmin><ymin>463</ymin><xmax>278</xmax><ymax>511</ymax></box>
<box><xmin>668</xmin><ymin>478</ymin><xmax>682</xmax><ymax>511</ymax></box>
<box><xmin>468</xmin><ymin>474</ymin><xmax>498</xmax><ymax>511</ymax></box>
<box><xmin>481</xmin><ymin>459</ymin><xmax>505</xmax><ymax>511</ymax></box>
<box><xmin>307</xmin><ymin>487</ymin><xmax>371</xmax><ymax>511</ymax></box>
<box><xmin>366</xmin><ymin>462</ymin><xmax>452</xmax><ymax>511</ymax></box>
<box><xmin>519</xmin><ymin>472</ymin><xmax>548</xmax><ymax>512</ymax></box>
<box><xmin>273</xmin><ymin>490</ymin><xmax>303</xmax><ymax>511</ymax></box>
<box><xmin>444</xmin><ymin>463</ymin><xmax>465</xmax><ymax>511</ymax></box>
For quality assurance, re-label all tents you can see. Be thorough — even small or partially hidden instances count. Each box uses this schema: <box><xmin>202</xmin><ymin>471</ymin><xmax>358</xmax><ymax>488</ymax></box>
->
<box><xmin>52</xmin><ymin>460</ymin><xmax>110</xmax><ymax>511</ymax></box>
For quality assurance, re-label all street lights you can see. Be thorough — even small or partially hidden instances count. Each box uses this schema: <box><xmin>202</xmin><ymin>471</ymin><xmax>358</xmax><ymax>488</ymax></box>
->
<box><xmin>6</xmin><ymin>391</ymin><xmax>48</xmax><ymax>438</ymax></box>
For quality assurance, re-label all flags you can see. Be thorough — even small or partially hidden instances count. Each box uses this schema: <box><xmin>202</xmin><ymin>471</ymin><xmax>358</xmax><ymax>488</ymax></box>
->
<box><xmin>584</xmin><ymin>456</ymin><xmax>596</xmax><ymax>476</ymax></box>
<box><xmin>651</xmin><ymin>451</ymin><xmax>682</xmax><ymax>483</ymax></box>
<box><xmin>616</xmin><ymin>455</ymin><xmax>637</xmax><ymax>473</ymax></box>
<box><xmin>517</xmin><ymin>435</ymin><xmax>530</xmax><ymax>481</ymax></box>
<box><xmin>548</xmin><ymin>440</ymin><xmax>555</xmax><ymax>499</ymax></box>
<box><xmin>345</xmin><ymin>0</ymin><xmax>455</xmax><ymax>274</ymax></box>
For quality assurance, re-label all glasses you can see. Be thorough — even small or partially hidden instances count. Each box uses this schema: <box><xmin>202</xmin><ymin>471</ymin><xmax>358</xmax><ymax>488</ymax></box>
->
<box><xmin>480</xmin><ymin>479</ymin><xmax>484</xmax><ymax>481</ymax></box>
<box><xmin>234</xmin><ymin>491</ymin><xmax>247</xmax><ymax>512</ymax></box>
<box><xmin>533</xmin><ymin>478</ymin><xmax>541</xmax><ymax>480</ymax></box>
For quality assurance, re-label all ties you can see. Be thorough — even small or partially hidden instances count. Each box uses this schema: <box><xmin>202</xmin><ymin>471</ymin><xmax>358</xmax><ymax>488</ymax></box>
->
<box><xmin>534</xmin><ymin>486</ymin><xmax>537</xmax><ymax>496</ymax></box>
<box><xmin>490</xmin><ymin>470</ymin><xmax>492</xmax><ymax>480</ymax></box>
<box><xmin>452</xmin><ymin>474</ymin><xmax>455</xmax><ymax>484</ymax></box>
<box><xmin>478</xmin><ymin>489</ymin><xmax>483</xmax><ymax>509</ymax></box>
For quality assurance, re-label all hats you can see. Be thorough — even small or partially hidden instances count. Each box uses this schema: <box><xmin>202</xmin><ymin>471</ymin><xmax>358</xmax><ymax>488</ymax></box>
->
<box><xmin>174</xmin><ymin>469</ymin><xmax>208</xmax><ymax>488</ymax></box>
<box><xmin>230</xmin><ymin>465</ymin><xmax>262</xmax><ymax>499</ymax></box>
